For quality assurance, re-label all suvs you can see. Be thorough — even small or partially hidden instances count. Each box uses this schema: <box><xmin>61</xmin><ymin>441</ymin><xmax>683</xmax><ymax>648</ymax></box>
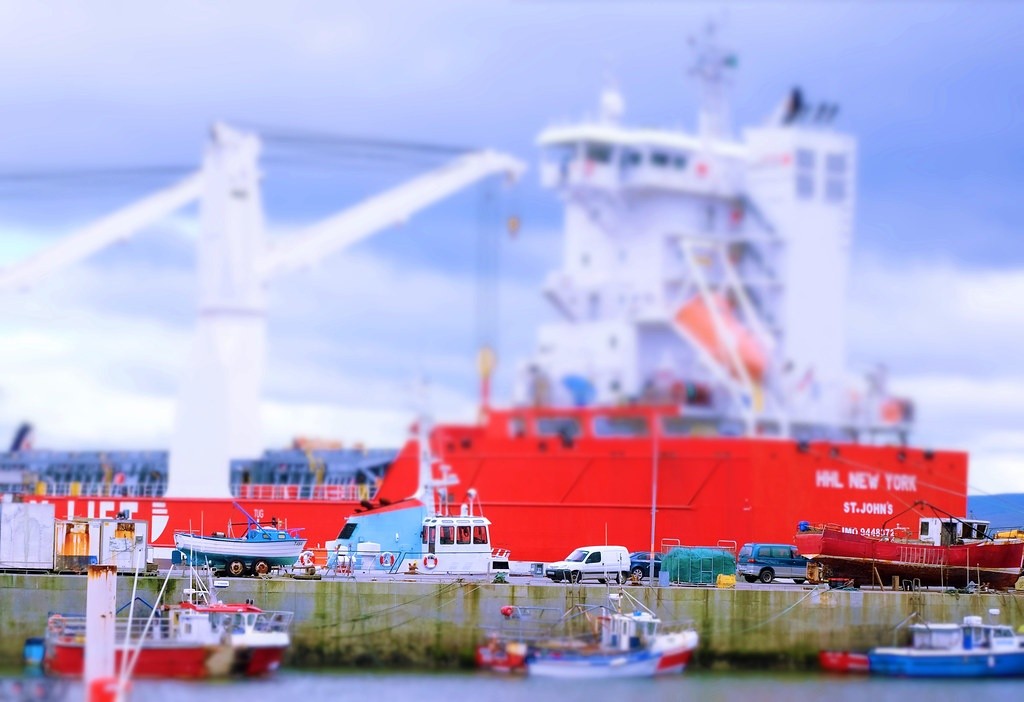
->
<box><xmin>546</xmin><ymin>546</ymin><xmax>631</xmax><ymax>584</ymax></box>
<box><xmin>737</xmin><ymin>542</ymin><xmax>811</xmax><ymax>584</ymax></box>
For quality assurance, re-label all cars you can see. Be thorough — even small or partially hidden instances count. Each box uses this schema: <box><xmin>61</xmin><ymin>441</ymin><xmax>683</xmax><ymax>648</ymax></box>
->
<box><xmin>628</xmin><ymin>551</ymin><xmax>665</xmax><ymax>581</ymax></box>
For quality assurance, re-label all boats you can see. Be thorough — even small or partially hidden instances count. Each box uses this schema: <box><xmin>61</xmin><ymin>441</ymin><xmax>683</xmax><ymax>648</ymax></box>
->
<box><xmin>44</xmin><ymin>510</ymin><xmax>293</xmax><ymax>681</ymax></box>
<box><xmin>817</xmin><ymin>547</ymin><xmax>1024</xmax><ymax>679</ymax></box>
<box><xmin>173</xmin><ymin>502</ymin><xmax>308</xmax><ymax>566</ymax></box>
<box><xmin>290</xmin><ymin>486</ymin><xmax>510</xmax><ymax>580</ymax></box>
<box><xmin>673</xmin><ymin>291</ymin><xmax>769</xmax><ymax>395</ymax></box>
<box><xmin>474</xmin><ymin>521</ymin><xmax>701</xmax><ymax>676</ymax></box>
<box><xmin>792</xmin><ymin>499</ymin><xmax>1024</xmax><ymax>592</ymax></box>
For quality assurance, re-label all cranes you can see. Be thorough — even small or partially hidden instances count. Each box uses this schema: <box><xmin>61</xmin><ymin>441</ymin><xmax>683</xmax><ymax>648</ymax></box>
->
<box><xmin>39</xmin><ymin>122</ymin><xmax>529</xmax><ymax>497</ymax></box>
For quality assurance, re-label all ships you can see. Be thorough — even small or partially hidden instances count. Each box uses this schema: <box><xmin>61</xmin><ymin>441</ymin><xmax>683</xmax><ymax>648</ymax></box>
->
<box><xmin>0</xmin><ymin>85</ymin><xmax>1024</xmax><ymax>572</ymax></box>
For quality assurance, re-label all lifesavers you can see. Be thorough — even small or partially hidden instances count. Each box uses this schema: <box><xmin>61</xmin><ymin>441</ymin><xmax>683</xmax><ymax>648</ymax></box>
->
<box><xmin>380</xmin><ymin>552</ymin><xmax>394</xmax><ymax>567</ymax></box>
<box><xmin>300</xmin><ymin>551</ymin><xmax>316</xmax><ymax>568</ymax></box>
<box><xmin>423</xmin><ymin>553</ymin><xmax>438</xmax><ymax>569</ymax></box>
